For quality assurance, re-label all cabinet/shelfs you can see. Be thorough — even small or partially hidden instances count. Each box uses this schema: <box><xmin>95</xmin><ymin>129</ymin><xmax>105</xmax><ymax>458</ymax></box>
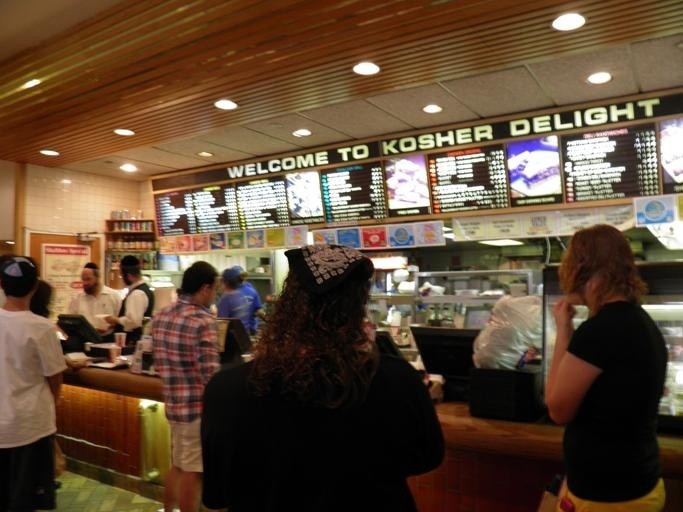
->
<box><xmin>106</xmin><ymin>219</ymin><xmax>159</xmax><ymax>289</ymax></box>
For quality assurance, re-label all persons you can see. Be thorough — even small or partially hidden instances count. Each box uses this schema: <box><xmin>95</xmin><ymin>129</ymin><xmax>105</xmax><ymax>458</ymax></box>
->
<box><xmin>540</xmin><ymin>223</ymin><xmax>670</xmax><ymax>512</ymax></box>
<box><xmin>28</xmin><ymin>279</ymin><xmax>53</xmax><ymax>317</ymax></box>
<box><xmin>469</xmin><ymin>288</ymin><xmax>556</xmax><ymax>416</ymax></box>
<box><xmin>211</xmin><ymin>268</ymin><xmax>251</xmax><ymax>342</ymax></box>
<box><xmin>103</xmin><ymin>255</ymin><xmax>156</xmax><ymax>348</ymax></box>
<box><xmin>65</xmin><ymin>262</ymin><xmax>125</xmax><ymax>341</ymax></box>
<box><xmin>0</xmin><ymin>253</ymin><xmax>70</xmax><ymax>510</ymax></box>
<box><xmin>232</xmin><ymin>266</ymin><xmax>270</xmax><ymax>336</ymax></box>
<box><xmin>147</xmin><ymin>261</ymin><xmax>225</xmax><ymax>512</ymax></box>
<box><xmin>197</xmin><ymin>242</ymin><xmax>447</xmax><ymax>511</ymax></box>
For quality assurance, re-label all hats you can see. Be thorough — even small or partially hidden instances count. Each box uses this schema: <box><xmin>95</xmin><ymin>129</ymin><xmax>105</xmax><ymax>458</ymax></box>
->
<box><xmin>284</xmin><ymin>244</ymin><xmax>374</xmax><ymax>294</ymax></box>
<box><xmin>222</xmin><ymin>266</ymin><xmax>243</xmax><ymax>279</ymax></box>
<box><xmin>2</xmin><ymin>256</ymin><xmax>36</xmax><ymax>278</ymax></box>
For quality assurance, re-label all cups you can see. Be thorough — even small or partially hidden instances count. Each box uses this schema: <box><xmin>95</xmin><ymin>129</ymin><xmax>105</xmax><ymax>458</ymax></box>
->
<box><xmin>114</xmin><ymin>332</ymin><xmax>127</xmax><ymax>346</ymax></box>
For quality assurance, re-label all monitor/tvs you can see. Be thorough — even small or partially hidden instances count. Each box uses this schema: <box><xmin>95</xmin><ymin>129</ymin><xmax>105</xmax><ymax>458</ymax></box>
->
<box><xmin>409</xmin><ymin>326</ymin><xmax>482</xmax><ymax>374</ymax></box>
<box><xmin>375</xmin><ymin>331</ymin><xmax>415</xmax><ymax>373</ymax></box>
<box><xmin>56</xmin><ymin>314</ymin><xmax>104</xmax><ymax>344</ymax></box>
<box><xmin>215</xmin><ymin>318</ymin><xmax>251</xmax><ymax>361</ymax></box>
